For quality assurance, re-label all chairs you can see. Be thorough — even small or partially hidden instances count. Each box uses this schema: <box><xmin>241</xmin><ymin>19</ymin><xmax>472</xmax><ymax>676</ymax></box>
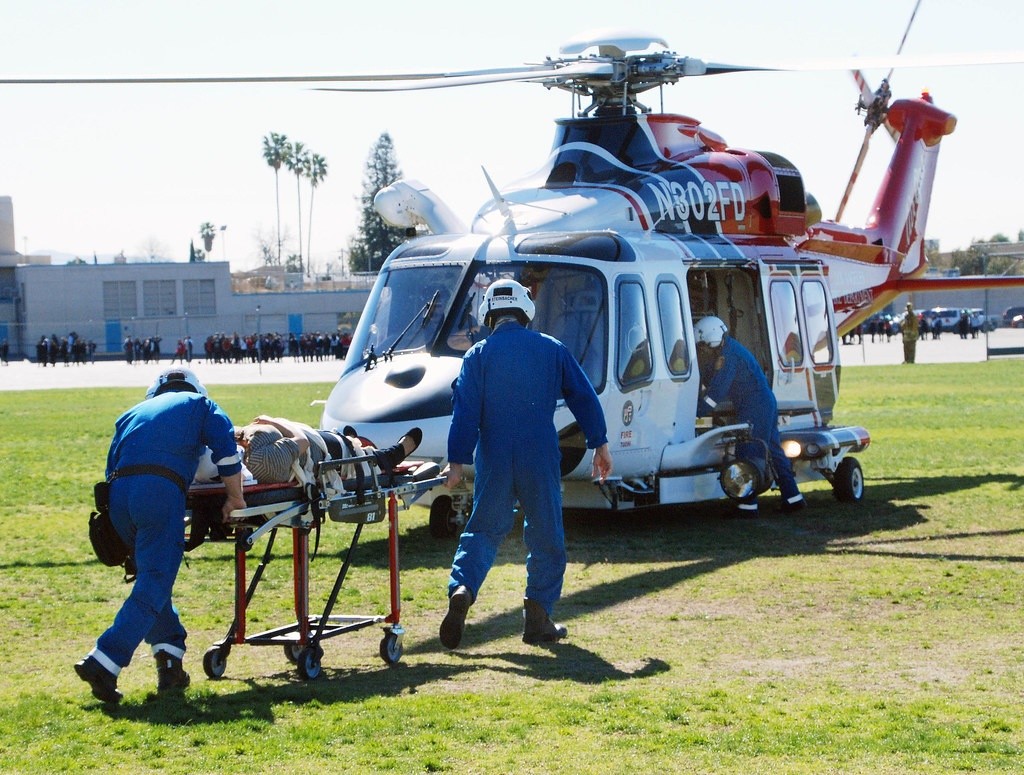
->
<box><xmin>550</xmin><ymin>290</ymin><xmax>606</xmax><ymax>388</ymax></box>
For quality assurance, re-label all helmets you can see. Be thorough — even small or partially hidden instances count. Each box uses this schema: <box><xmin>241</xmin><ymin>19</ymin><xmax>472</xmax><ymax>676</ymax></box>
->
<box><xmin>694</xmin><ymin>316</ymin><xmax>728</xmax><ymax>348</ymax></box>
<box><xmin>146</xmin><ymin>369</ymin><xmax>208</xmax><ymax>398</ymax></box>
<box><xmin>477</xmin><ymin>279</ymin><xmax>536</xmax><ymax>330</ymax></box>
<box><xmin>192</xmin><ymin>445</ymin><xmax>245</xmax><ymax>485</ymax></box>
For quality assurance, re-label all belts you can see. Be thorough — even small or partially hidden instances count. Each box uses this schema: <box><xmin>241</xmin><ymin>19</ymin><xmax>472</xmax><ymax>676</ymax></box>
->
<box><xmin>106</xmin><ymin>464</ymin><xmax>185</xmax><ymax>492</ymax></box>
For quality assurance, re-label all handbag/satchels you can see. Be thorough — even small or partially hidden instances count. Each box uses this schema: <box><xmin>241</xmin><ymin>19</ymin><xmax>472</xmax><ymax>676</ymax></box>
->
<box><xmin>88</xmin><ymin>481</ymin><xmax>129</xmax><ymax>569</ymax></box>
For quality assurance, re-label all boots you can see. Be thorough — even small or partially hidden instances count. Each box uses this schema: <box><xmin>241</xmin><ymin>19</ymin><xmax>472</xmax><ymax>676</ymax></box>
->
<box><xmin>154</xmin><ymin>649</ymin><xmax>190</xmax><ymax>693</ymax></box>
<box><xmin>440</xmin><ymin>586</ymin><xmax>472</xmax><ymax>649</ymax></box>
<box><xmin>75</xmin><ymin>658</ymin><xmax>124</xmax><ymax>702</ymax></box>
<box><xmin>521</xmin><ymin>596</ymin><xmax>568</xmax><ymax>643</ymax></box>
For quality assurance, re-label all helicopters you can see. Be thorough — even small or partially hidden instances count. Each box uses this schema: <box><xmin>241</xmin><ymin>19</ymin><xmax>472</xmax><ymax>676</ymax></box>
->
<box><xmin>0</xmin><ymin>32</ymin><xmax>1024</xmax><ymax>534</ymax></box>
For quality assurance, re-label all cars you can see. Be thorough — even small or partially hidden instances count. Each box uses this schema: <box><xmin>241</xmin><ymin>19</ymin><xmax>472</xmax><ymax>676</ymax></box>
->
<box><xmin>1002</xmin><ymin>306</ymin><xmax>1024</xmax><ymax>328</ymax></box>
<box><xmin>859</xmin><ymin>307</ymin><xmax>997</xmax><ymax>334</ymax></box>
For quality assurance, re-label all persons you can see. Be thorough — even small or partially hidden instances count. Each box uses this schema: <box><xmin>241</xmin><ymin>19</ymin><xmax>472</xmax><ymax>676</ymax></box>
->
<box><xmin>694</xmin><ymin>316</ymin><xmax>807</xmax><ymax>520</ymax></box>
<box><xmin>203</xmin><ymin>331</ymin><xmax>286</xmax><ymax>365</ymax></box>
<box><xmin>869</xmin><ymin>319</ymin><xmax>891</xmax><ymax>343</ymax></box>
<box><xmin>72</xmin><ymin>365</ymin><xmax>247</xmax><ymax>707</ymax></box>
<box><xmin>439</xmin><ymin>279</ymin><xmax>614</xmax><ymax>650</ymax></box>
<box><xmin>901</xmin><ymin>303</ymin><xmax>918</xmax><ymax>364</ymax></box>
<box><xmin>123</xmin><ymin>335</ymin><xmax>162</xmax><ymax>364</ymax></box>
<box><xmin>842</xmin><ymin>325</ymin><xmax>861</xmax><ymax>345</ymax></box>
<box><xmin>919</xmin><ymin>312</ymin><xmax>941</xmax><ymax>340</ymax></box>
<box><xmin>37</xmin><ymin>331</ymin><xmax>97</xmax><ymax>368</ymax></box>
<box><xmin>287</xmin><ymin>332</ymin><xmax>352</xmax><ymax>363</ymax></box>
<box><xmin>0</xmin><ymin>338</ymin><xmax>9</xmax><ymax>366</ymax></box>
<box><xmin>171</xmin><ymin>337</ymin><xmax>193</xmax><ymax>365</ymax></box>
<box><xmin>232</xmin><ymin>415</ymin><xmax>422</xmax><ymax>484</ymax></box>
<box><xmin>958</xmin><ymin>312</ymin><xmax>980</xmax><ymax>339</ymax></box>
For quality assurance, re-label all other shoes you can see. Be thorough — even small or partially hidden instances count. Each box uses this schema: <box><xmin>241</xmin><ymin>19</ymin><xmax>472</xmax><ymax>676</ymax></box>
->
<box><xmin>718</xmin><ymin>506</ymin><xmax>759</xmax><ymax>519</ymax></box>
<box><xmin>396</xmin><ymin>427</ymin><xmax>423</xmax><ymax>465</ymax></box>
<box><xmin>777</xmin><ymin>500</ymin><xmax>808</xmax><ymax>515</ymax></box>
<box><xmin>343</xmin><ymin>426</ymin><xmax>358</xmax><ymax>439</ymax></box>
